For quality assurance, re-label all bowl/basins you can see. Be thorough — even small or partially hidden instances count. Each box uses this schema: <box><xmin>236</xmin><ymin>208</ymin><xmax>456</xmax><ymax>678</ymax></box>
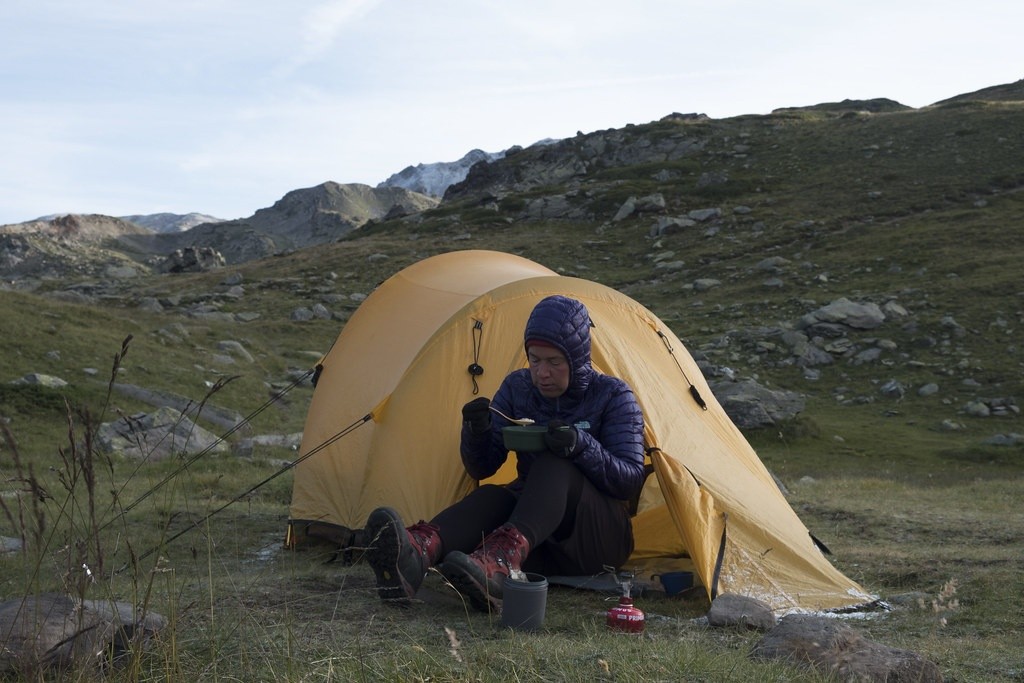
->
<box><xmin>502</xmin><ymin>425</ymin><xmax>552</xmax><ymax>451</ymax></box>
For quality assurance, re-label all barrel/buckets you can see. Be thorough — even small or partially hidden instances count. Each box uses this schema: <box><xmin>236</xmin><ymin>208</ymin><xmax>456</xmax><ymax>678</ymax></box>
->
<box><xmin>502</xmin><ymin>573</ymin><xmax>549</xmax><ymax>631</ymax></box>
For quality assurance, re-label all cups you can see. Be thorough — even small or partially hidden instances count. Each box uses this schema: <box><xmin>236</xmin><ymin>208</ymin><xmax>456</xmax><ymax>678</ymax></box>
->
<box><xmin>650</xmin><ymin>571</ymin><xmax>694</xmax><ymax>594</ymax></box>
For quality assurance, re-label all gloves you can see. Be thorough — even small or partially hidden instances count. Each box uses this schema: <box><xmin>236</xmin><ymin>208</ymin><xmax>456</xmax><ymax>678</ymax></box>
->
<box><xmin>462</xmin><ymin>397</ymin><xmax>494</xmax><ymax>434</ymax></box>
<box><xmin>543</xmin><ymin>420</ymin><xmax>577</xmax><ymax>458</ymax></box>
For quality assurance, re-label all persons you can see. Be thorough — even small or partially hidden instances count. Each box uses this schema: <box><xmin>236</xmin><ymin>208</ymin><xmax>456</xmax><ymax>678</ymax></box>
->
<box><xmin>362</xmin><ymin>295</ymin><xmax>645</xmax><ymax>616</ymax></box>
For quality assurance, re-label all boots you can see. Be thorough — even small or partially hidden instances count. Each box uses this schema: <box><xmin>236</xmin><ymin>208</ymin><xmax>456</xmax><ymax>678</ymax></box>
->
<box><xmin>362</xmin><ymin>507</ymin><xmax>443</xmax><ymax>611</ymax></box>
<box><xmin>440</xmin><ymin>521</ymin><xmax>531</xmax><ymax>615</ymax></box>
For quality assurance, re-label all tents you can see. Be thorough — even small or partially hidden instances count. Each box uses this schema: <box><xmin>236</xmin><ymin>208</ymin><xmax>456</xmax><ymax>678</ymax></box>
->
<box><xmin>287</xmin><ymin>250</ymin><xmax>888</xmax><ymax>623</ymax></box>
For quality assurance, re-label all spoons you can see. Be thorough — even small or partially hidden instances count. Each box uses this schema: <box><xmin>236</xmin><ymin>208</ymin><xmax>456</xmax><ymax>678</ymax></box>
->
<box><xmin>488</xmin><ymin>406</ymin><xmax>535</xmax><ymax>425</ymax></box>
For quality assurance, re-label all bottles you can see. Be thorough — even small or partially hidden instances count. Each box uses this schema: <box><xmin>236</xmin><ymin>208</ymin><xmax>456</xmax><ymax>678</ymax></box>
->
<box><xmin>607</xmin><ymin>596</ymin><xmax>644</xmax><ymax>633</ymax></box>
